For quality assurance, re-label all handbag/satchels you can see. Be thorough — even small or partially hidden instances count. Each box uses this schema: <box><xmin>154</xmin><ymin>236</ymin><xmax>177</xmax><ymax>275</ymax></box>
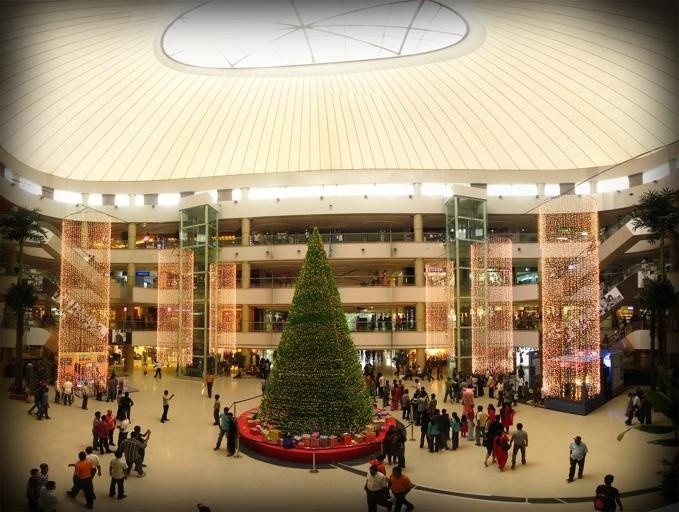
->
<box><xmin>504</xmin><ymin>443</ymin><xmax>511</xmax><ymax>450</ymax></box>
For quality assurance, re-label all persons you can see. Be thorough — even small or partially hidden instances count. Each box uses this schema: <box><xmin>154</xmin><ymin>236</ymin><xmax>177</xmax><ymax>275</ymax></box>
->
<box><xmin>25</xmin><ymin>348</ymin><xmax>160</xmax><ymax>424</ymax></box>
<box><xmin>623</xmin><ymin>386</ymin><xmax>654</xmax><ymax>427</ymax></box>
<box><xmin>599</xmin><ymin>209</ymin><xmax>648</xmax><ymax>349</ymax></box>
<box><xmin>126</xmin><ymin>313</ymin><xmax>157</xmax><ymax>331</ymax></box>
<box><xmin>355</xmin><ymin>314</ymin><xmax>407</xmax><ymax>332</ymax></box>
<box><xmin>206</xmin><ymin>372</ymin><xmax>213</xmax><ymax>396</ymax></box>
<box><xmin>275</xmin><ymin>270</ymin><xmax>296</xmax><ymax>288</ymax></box>
<box><xmin>251</xmin><ymin>229</ymin><xmax>345</xmax><ymax>245</ymax></box>
<box><xmin>213</xmin><ymin>394</ymin><xmax>237</xmax><ymax>456</ymax></box>
<box><xmin>115</xmin><ymin>328</ymin><xmax>123</xmax><ymax>343</ymax></box>
<box><xmin>26</xmin><ymin>409</ymin><xmax>151</xmax><ymax>511</ymax></box>
<box><xmin>159</xmin><ymin>390</ymin><xmax>174</xmax><ymax>422</ymax></box>
<box><xmin>371</xmin><ymin>269</ymin><xmax>388</xmax><ymax>285</ymax></box>
<box><xmin>220</xmin><ymin>350</ymin><xmax>270</xmax><ymax>379</ymax></box>
<box><xmin>566</xmin><ymin>435</ymin><xmax>588</xmax><ymax>482</ymax></box>
<box><xmin>270</xmin><ymin>315</ymin><xmax>286</xmax><ymax>331</ymax></box>
<box><xmin>594</xmin><ymin>474</ymin><xmax>624</xmax><ymax>512</ymax></box>
<box><xmin>380</xmin><ymin>228</ymin><xmax>386</xmax><ymax>241</ymax></box>
<box><xmin>360</xmin><ymin>348</ymin><xmax>543</xmax><ymax>511</ymax></box>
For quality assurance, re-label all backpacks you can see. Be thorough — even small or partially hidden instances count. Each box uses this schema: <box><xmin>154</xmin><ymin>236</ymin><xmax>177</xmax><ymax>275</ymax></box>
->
<box><xmin>425</xmin><ymin>398</ymin><xmax>430</xmax><ymax>410</ymax></box>
<box><xmin>593</xmin><ymin>493</ymin><xmax>610</xmax><ymax>511</ymax></box>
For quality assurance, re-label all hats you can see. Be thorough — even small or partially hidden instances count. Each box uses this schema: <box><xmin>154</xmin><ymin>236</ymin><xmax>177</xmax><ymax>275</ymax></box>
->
<box><xmin>573</xmin><ymin>436</ymin><xmax>581</xmax><ymax>441</ymax></box>
<box><xmin>447</xmin><ymin>377</ymin><xmax>453</xmax><ymax>380</ymax></box>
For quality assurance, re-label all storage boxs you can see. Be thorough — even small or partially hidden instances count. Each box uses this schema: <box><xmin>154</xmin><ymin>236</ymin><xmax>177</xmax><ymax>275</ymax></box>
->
<box><xmin>245</xmin><ymin>400</ymin><xmax>394</xmax><ymax>451</ymax></box>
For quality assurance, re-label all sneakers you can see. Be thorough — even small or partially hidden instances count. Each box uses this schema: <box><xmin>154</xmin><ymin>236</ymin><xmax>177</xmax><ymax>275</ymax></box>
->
<box><xmin>387</xmin><ymin>461</ymin><xmax>405</xmax><ymax>473</ymax></box>
<box><xmin>92</xmin><ymin>441</ymin><xmax>117</xmax><ymax>455</ymax></box>
<box><xmin>85</xmin><ymin>503</ymin><xmax>93</xmax><ymax>509</ymax></box>
<box><xmin>109</xmin><ymin>463</ymin><xmax>149</xmax><ymax>500</ymax></box>
<box><xmin>92</xmin><ymin>493</ymin><xmax>97</xmax><ymax>500</ymax></box>
<box><xmin>67</xmin><ymin>487</ymin><xmax>80</xmax><ymax>497</ymax></box>
<box><xmin>461</xmin><ymin>435</ymin><xmax>487</xmax><ymax>447</ymax></box>
<box><xmin>385</xmin><ymin>496</ymin><xmax>392</xmax><ymax>499</ymax></box>
<box><xmin>402</xmin><ymin>415</ymin><xmax>422</xmax><ymax>426</ymax></box>
<box><xmin>419</xmin><ymin>443</ymin><xmax>458</xmax><ymax>453</ymax></box>
<box><xmin>28</xmin><ymin>410</ymin><xmax>51</xmax><ymax>421</ymax></box>
<box><xmin>160</xmin><ymin>418</ymin><xmax>170</xmax><ymax>423</ymax></box>
<box><xmin>483</xmin><ymin>459</ymin><xmax>585</xmax><ymax>483</ymax></box>
<box><xmin>405</xmin><ymin>505</ymin><xmax>414</xmax><ymax>511</ymax></box>
<box><xmin>211</xmin><ymin>422</ymin><xmax>235</xmax><ymax>457</ymax></box>
<box><xmin>387</xmin><ymin>502</ymin><xmax>393</xmax><ymax>511</ymax></box>
